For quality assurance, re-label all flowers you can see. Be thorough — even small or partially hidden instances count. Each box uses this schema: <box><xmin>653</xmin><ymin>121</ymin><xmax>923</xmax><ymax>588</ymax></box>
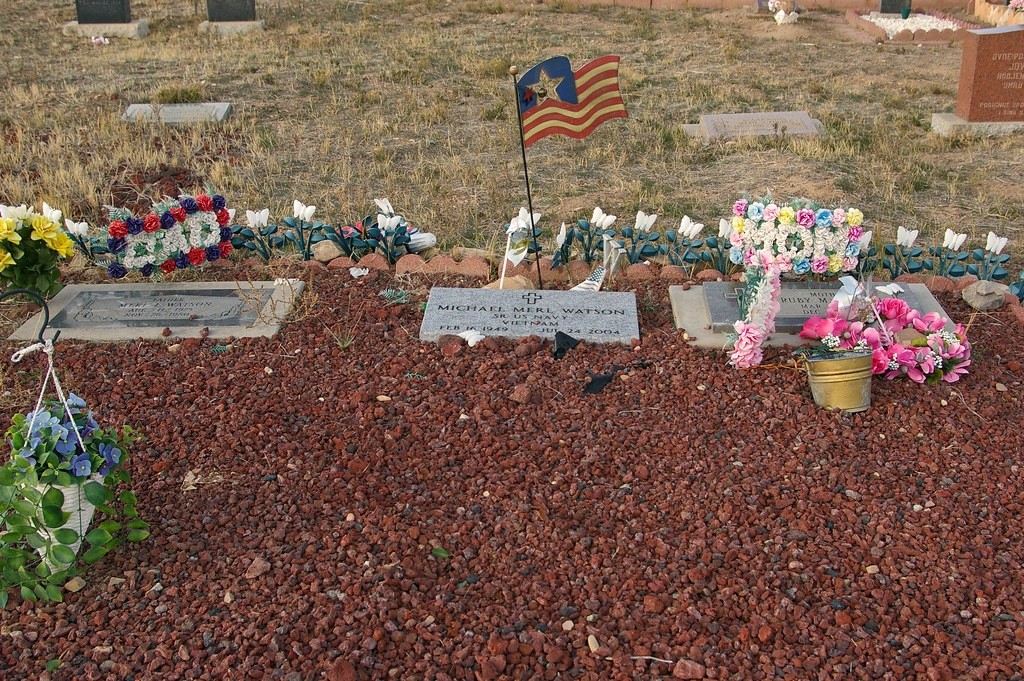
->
<box><xmin>0</xmin><ymin>192</ymin><xmax>419</xmax><ymax>304</ymax></box>
<box><xmin>0</xmin><ymin>393</ymin><xmax>151</xmax><ymax>609</ymax></box>
<box><xmin>508</xmin><ymin>195</ymin><xmax>1011</xmax><ymax>385</ymax></box>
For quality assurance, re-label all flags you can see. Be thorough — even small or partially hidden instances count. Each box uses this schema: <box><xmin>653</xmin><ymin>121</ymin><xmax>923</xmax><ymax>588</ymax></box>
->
<box><xmin>515</xmin><ymin>54</ymin><xmax>629</xmax><ymax>147</ymax></box>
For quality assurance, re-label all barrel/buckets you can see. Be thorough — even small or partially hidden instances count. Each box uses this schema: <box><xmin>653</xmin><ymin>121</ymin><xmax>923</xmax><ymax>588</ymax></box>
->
<box><xmin>802</xmin><ymin>344</ymin><xmax>873</xmax><ymax>414</ymax></box>
